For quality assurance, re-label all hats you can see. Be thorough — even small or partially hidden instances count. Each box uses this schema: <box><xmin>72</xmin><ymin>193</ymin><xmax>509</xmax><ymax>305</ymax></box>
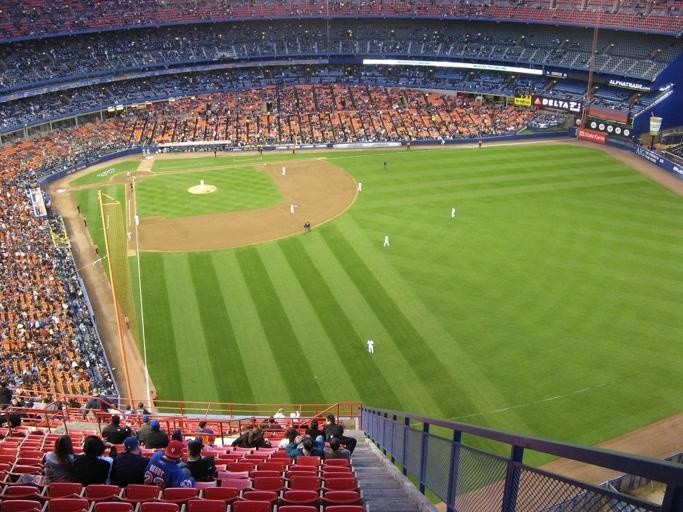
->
<box><xmin>120</xmin><ymin>436</ymin><xmax>140</xmax><ymax>454</ymax></box>
<box><xmin>81</xmin><ymin>435</ymin><xmax>105</xmax><ymax>457</ymax></box>
<box><xmin>163</xmin><ymin>438</ymin><xmax>184</xmax><ymax>459</ymax></box>
<box><xmin>107</xmin><ymin>413</ymin><xmax>208</xmax><ymax>437</ymax></box>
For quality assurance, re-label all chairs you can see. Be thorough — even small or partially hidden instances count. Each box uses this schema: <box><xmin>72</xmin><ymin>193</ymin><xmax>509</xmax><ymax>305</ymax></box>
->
<box><xmin>1</xmin><ymin>405</ymin><xmax>366</xmax><ymax>511</ymax></box>
